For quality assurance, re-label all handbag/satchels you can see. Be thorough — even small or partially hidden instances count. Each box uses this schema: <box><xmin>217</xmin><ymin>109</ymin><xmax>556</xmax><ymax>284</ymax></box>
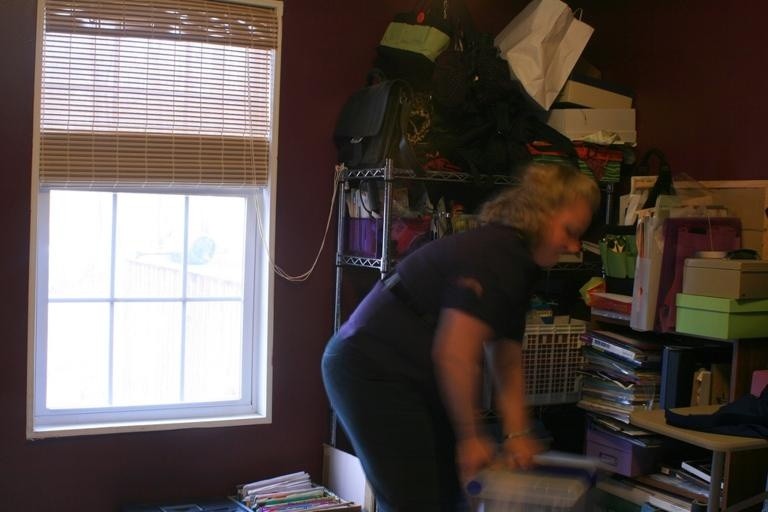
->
<box><xmin>333</xmin><ymin>80</ymin><xmax>413</xmax><ymax>163</ymax></box>
<box><xmin>493</xmin><ymin>0</ymin><xmax>594</xmax><ymax>110</ymax></box>
<box><xmin>376</xmin><ymin>9</ymin><xmax>451</xmax><ymax>71</ymax></box>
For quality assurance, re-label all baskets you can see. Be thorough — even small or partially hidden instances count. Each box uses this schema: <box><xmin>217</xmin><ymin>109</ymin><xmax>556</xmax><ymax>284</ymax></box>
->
<box><xmin>521</xmin><ymin>318</ymin><xmax>585</xmax><ymax>406</ymax></box>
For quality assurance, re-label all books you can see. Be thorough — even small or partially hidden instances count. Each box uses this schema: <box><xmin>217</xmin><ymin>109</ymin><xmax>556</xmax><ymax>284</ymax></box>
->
<box><xmin>235</xmin><ymin>468</ymin><xmax>354</xmax><ymax>512</ymax></box>
<box><xmin>344</xmin><ymin>187</ymin><xmax>410</xmax><ymax>260</ymax></box>
<box><xmin>580</xmin><ymin>177</ymin><xmax>728</xmax><ymax>512</ymax></box>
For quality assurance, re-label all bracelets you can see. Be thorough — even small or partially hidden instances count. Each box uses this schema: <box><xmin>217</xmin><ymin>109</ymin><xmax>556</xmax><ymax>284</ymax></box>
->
<box><xmin>499</xmin><ymin>425</ymin><xmax>541</xmax><ymax>442</ymax></box>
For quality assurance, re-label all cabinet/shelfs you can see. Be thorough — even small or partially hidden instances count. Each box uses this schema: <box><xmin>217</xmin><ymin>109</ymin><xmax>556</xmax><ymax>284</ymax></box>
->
<box><xmin>576</xmin><ymin>176</ymin><xmax>767</xmax><ymax>512</ymax></box>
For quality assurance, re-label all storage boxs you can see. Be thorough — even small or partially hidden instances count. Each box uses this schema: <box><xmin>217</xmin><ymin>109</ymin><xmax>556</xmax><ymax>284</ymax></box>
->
<box><xmin>545</xmin><ymin>78</ymin><xmax>637</xmax><ymax>145</ymax></box>
<box><xmin>584</xmin><ymin>427</ymin><xmax>668</xmax><ymax>478</ymax></box>
<box><xmin>673</xmin><ymin>253</ymin><xmax>766</xmax><ymax>341</ymax></box>
<box><xmin>346</xmin><ymin>217</ymin><xmax>429</xmax><ymax>259</ymax></box>
<box><xmin>464</xmin><ymin>456</ymin><xmax>596</xmax><ymax>511</ymax></box>
<box><xmin>227</xmin><ymin>484</ymin><xmax>362</xmax><ymax>512</ymax></box>
